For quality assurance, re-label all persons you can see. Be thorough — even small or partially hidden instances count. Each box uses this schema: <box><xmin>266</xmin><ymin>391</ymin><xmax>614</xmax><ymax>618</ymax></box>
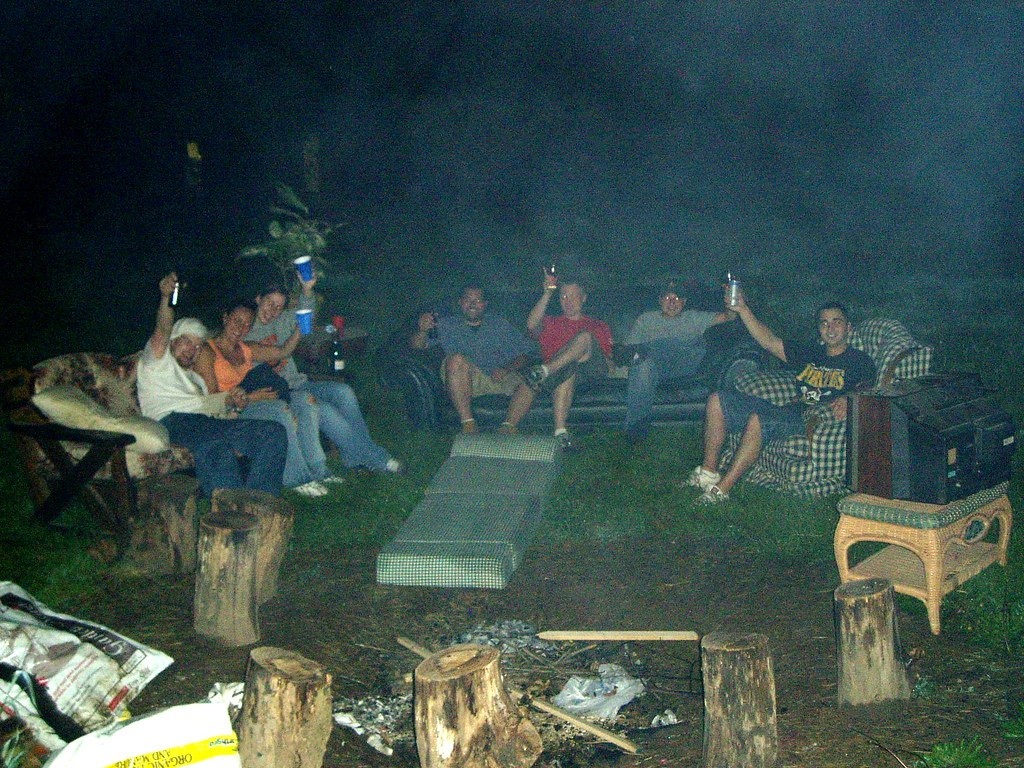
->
<box><xmin>682</xmin><ymin>286</ymin><xmax>878</xmax><ymax>508</ymax></box>
<box><xmin>517</xmin><ymin>263</ymin><xmax>613</xmax><ymax>455</ymax></box>
<box><xmin>411</xmin><ymin>282</ymin><xmax>540</xmax><ymax>437</ymax></box>
<box><xmin>620</xmin><ymin>277</ymin><xmax>743</xmax><ymax>448</ymax></box>
<box><xmin>241</xmin><ymin>270</ymin><xmax>404</xmax><ymax>473</ymax></box>
<box><xmin>137</xmin><ymin>271</ymin><xmax>287</xmax><ymax>493</ymax></box>
<box><xmin>193</xmin><ymin>297</ymin><xmax>344</xmax><ymax>497</ymax></box>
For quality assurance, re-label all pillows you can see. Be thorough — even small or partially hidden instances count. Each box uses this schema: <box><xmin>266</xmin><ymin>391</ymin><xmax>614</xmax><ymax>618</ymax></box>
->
<box><xmin>28</xmin><ymin>384</ymin><xmax>172</xmax><ymax>455</ymax></box>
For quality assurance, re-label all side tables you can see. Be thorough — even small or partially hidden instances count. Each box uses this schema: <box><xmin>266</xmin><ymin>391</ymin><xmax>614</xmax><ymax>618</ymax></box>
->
<box><xmin>295</xmin><ymin>325</ymin><xmax>368</xmax><ymax>382</ymax></box>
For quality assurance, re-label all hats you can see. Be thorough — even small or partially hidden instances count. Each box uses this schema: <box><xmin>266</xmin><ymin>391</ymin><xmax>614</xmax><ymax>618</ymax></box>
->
<box><xmin>660</xmin><ymin>282</ymin><xmax>685</xmax><ymax>298</ymax></box>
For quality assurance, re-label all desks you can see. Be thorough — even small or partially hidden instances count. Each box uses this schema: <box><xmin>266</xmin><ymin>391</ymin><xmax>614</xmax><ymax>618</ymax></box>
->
<box><xmin>833</xmin><ymin>480</ymin><xmax>1014</xmax><ymax>636</ymax></box>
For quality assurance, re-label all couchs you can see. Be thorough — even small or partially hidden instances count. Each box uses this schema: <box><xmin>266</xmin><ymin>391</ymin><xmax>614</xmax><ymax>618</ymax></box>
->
<box><xmin>375</xmin><ymin>281</ymin><xmax>764</xmax><ymax>433</ymax></box>
<box><xmin>714</xmin><ymin>318</ymin><xmax>931</xmax><ymax>500</ymax></box>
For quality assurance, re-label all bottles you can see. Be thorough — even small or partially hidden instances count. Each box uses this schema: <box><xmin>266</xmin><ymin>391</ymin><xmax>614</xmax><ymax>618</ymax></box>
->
<box><xmin>330</xmin><ymin>340</ymin><xmax>345</xmax><ymax>376</ymax></box>
<box><xmin>429</xmin><ymin>310</ymin><xmax>438</xmax><ymax>339</ymax></box>
<box><xmin>729</xmin><ymin>276</ymin><xmax>741</xmax><ymax>306</ymax></box>
<box><xmin>723</xmin><ymin>269</ymin><xmax>732</xmax><ymax>302</ymax></box>
<box><xmin>168</xmin><ymin>277</ymin><xmax>183</xmax><ymax>308</ymax></box>
<box><xmin>548</xmin><ymin>264</ymin><xmax>557</xmax><ymax>289</ymax></box>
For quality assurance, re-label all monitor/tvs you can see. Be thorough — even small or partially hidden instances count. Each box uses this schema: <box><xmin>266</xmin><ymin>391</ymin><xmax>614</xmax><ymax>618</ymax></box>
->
<box><xmin>845</xmin><ymin>371</ymin><xmax>1016</xmax><ymax>505</ymax></box>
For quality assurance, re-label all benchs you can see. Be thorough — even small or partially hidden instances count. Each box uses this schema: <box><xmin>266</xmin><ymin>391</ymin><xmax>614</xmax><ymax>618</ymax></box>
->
<box><xmin>375</xmin><ymin>433</ymin><xmax>561</xmax><ymax>589</ymax></box>
<box><xmin>0</xmin><ymin>350</ymin><xmax>353</xmax><ymax>563</ymax></box>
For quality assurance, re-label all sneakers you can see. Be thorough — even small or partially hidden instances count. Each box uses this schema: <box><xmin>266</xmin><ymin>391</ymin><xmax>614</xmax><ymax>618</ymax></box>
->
<box><xmin>678</xmin><ymin>465</ymin><xmax>722</xmax><ymax>489</ymax></box>
<box><xmin>692</xmin><ymin>485</ymin><xmax>729</xmax><ymax>510</ymax></box>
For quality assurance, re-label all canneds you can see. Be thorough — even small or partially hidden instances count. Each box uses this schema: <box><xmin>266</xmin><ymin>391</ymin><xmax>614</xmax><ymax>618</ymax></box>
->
<box><xmin>729</xmin><ymin>280</ymin><xmax>740</xmax><ymax>306</ymax></box>
<box><xmin>169</xmin><ymin>281</ymin><xmax>182</xmax><ymax>306</ymax></box>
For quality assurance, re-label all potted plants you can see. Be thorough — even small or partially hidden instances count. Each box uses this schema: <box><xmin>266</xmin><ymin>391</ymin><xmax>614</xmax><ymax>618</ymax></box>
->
<box><xmin>231</xmin><ymin>186</ymin><xmax>349</xmax><ymax>332</ymax></box>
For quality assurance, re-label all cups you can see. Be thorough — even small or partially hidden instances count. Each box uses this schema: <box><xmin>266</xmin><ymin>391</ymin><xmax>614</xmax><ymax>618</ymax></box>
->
<box><xmin>295</xmin><ymin>309</ymin><xmax>313</xmax><ymax>335</ymax></box>
<box><xmin>294</xmin><ymin>255</ymin><xmax>313</xmax><ymax>282</ymax></box>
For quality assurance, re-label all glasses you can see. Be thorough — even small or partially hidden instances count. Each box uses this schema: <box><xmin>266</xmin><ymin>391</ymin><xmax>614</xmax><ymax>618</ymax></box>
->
<box><xmin>663</xmin><ymin>296</ymin><xmax>680</xmax><ymax>300</ymax></box>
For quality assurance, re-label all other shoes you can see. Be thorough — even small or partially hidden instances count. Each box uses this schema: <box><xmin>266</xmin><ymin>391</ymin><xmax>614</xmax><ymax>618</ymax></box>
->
<box><xmin>517</xmin><ymin>367</ymin><xmax>553</xmax><ymax>396</ymax></box>
<box><xmin>350</xmin><ymin>467</ymin><xmax>372</xmax><ymax>480</ymax></box>
<box><xmin>292</xmin><ymin>479</ymin><xmax>327</xmax><ymax>498</ymax></box>
<box><xmin>612</xmin><ymin>344</ymin><xmax>637</xmax><ymax>369</ymax></box>
<box><xmin>462</xmin><ymin>422</ymin><xmax>479</xmax><ymax>434</ymax></box>
<box><xmin>323</xmin><ymin>475</ymin><xmax>346</xmax><ymax>485</ymax></box>
<box><xmin>495</xmin><ymin>424</ymin><xmax>518</xmax><ymax>435</ymax></box>
<box><xmin>393</xmin><ymin>458</ymin><xmax>413</xmax><ymax>476</ymax></box>
<box><xmin>627</xmin><ymin>426</ymin><xmax>646</xmax><ymax>445</ymax></box>
<box><xmin>554</xmin><ymin>430</ymin><xmax>582</xmax><ymax>455</ymax></box>
